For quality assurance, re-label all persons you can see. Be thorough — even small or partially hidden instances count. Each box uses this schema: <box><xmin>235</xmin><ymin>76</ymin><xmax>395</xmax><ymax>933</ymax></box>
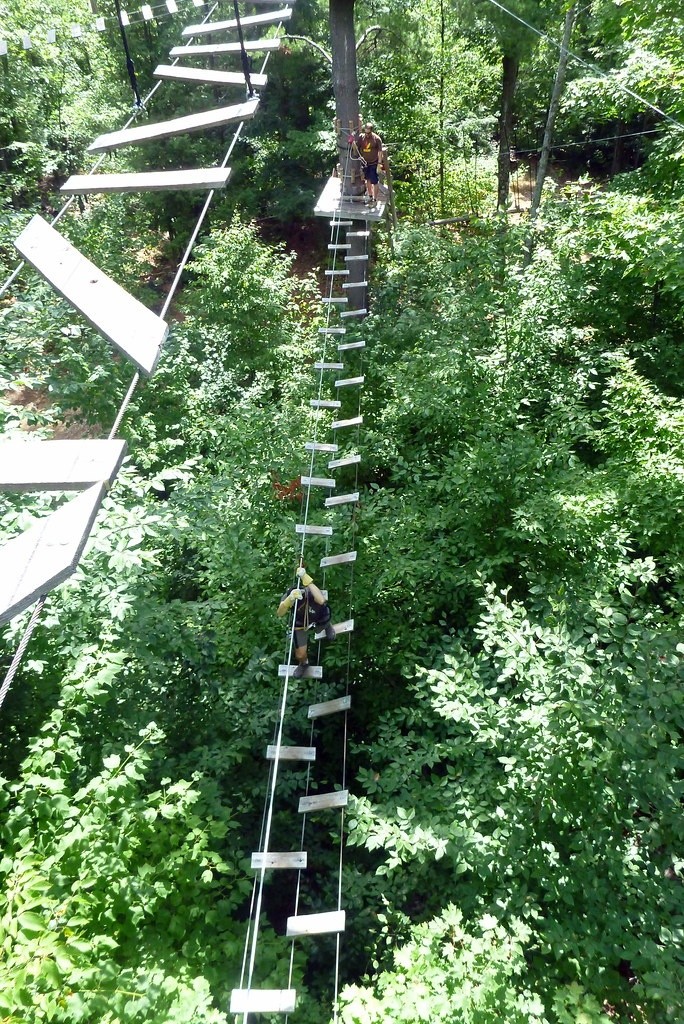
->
<box><xmin>350</xmin><ymin>122</ymin><xmax>385</xmax><ymax>210</ymax></box>
<box><xmin>276</xmin><ymin>568</ymin><xmax>336</xmax><ymax>680</ymax></box>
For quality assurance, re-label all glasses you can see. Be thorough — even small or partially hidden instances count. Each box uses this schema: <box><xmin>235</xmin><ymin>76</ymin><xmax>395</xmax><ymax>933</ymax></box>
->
<box><xmin>364</xmin><ymin>130</ymin><xmax>373</xmax><ymax>135</ymax></box>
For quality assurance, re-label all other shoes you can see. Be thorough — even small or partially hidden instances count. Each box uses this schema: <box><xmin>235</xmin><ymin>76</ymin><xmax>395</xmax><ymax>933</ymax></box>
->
<box><xmin>368</xmin><ymin>201</ymin><xmax>377</xmax><ymax>209</ymax></box>
<box><xmin>365</xmin><ymin>197</ymin><xmax>373</xmax><ymax>204</ymax></box>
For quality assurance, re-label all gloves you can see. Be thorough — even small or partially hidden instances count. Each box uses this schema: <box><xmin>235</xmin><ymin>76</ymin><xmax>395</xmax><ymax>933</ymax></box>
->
<box><xmin>283</xmin><ymin>589</ymin><xmax>305</xmax><ymax>608</ymax></box>
<box><xmin>296</xmin><ymin>567</ymin><xmax>314</xmax><ymax>585</ymax></box>
<box><xmin>376</xmin><ymin>164</ymin><xmax>382</xmax><ymax>175</ymax></box>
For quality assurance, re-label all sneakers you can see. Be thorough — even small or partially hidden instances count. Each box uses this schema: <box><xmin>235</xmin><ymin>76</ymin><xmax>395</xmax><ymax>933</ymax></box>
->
<box><xmin>325</xmin><ymin>622</ymin><xmax>336</xmax><ymax>640</ymax></box>
<box><xmin>292</xmin><ymin>657</ymin><xmax>310</xmax><ymax>679</ymax></box>
